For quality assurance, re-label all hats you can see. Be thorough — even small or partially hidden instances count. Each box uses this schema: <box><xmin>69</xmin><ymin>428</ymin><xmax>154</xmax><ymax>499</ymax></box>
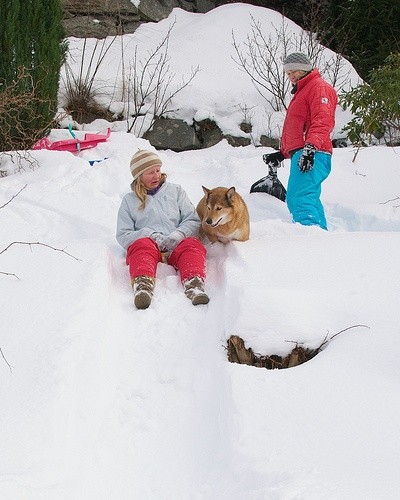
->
<box><xmin>283</xmin><ymin>52</ymin><xmax>313</xmax><ymax>72</ymax></box>
<box><xmin>129</xmin><ymin>147</ymin><xmax>162</xmax><ymax>180</ymax></box>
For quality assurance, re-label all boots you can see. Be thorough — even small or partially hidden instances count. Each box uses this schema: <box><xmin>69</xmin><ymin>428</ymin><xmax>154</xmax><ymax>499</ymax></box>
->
<box><xmin>131</xmin><ymin>275</ymin><xmax>155</xmax><ymax>310</ymax></box>
<box><xmin>182</xmin><ymin>276</ymin><xmax>210</xmax><ymax>306</ymax></box>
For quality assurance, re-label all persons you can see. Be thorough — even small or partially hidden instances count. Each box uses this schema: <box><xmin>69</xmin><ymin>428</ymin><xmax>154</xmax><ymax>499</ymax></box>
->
<box><xmin>116</xmin><ymin>150</ymin><xmax>210</xmax><ymax>309</ymax></box>
<box><xmin>279</xmin><ymin>52</ymin><xmax>339</xmax><ymax>231</ymax></box>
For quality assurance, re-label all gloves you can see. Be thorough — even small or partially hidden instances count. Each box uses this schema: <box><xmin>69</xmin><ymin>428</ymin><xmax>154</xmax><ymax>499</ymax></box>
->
<box><xmin>159</xmin><ymin>231</ymin><xmax>184</xmax><ymax>251</ymax></box>
<box><xmin>151</xmin><ymin>232</ymin><xmax>171</xmax><ymax>251</ymax></box>
<box><xmin>297</xmin><ymin>143</ymin><xmax>316</xmax><ymax>173</ymax></box>
<box><xmin>262</xmin><ymin>151</ymin><xmax>286</xmax><ymax>167</ymax></box>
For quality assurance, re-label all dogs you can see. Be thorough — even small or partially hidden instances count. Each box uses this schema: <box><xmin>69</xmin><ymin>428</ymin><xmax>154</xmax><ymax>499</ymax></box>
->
<box><xmin>194</xmin><ymin>185</ymin><xmax>250</xmax><ymax>246</ymax></box>
